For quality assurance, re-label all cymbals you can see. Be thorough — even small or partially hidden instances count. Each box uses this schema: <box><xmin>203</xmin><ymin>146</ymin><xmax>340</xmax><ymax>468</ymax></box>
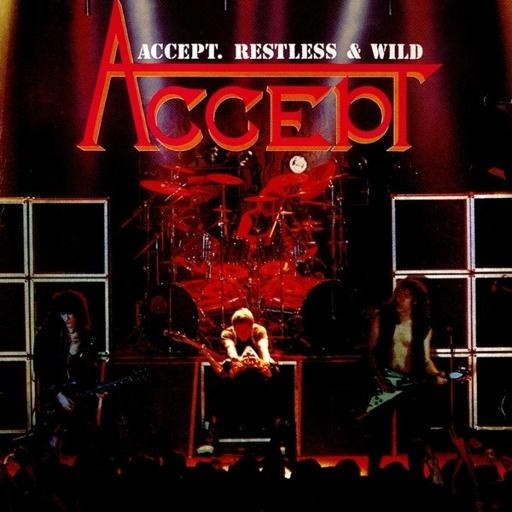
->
<box><xmin>299</xmin><ymin>160</ymin><xmax>348</xmax><ymax>197</ymax></box>
<box><xmin>244</xmin><ymin>197</ymin><xmax>278</xmax><ymax>202</ymax></box>
<box><xmin>142</xmin><ymin>161</ymin><xmax>242</xmax><ymax>198</ymax></box>
<box><xmin>292</xmin><ymin>220</ymin><xmax>322</xmax><ymax>233</ymax></box>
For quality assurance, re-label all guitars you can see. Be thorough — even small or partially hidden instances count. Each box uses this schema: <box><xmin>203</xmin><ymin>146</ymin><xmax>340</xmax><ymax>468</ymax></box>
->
<box><xmin>366</xmin><ymin>368</ymin><xmax>472</xmax><ymax>413</ymax></box>
<box><xmin>163</xmin><ymin>330</ymin><xmax>237</xmax><ymax>366</ymax></box>
<box><xmin>47</xmin><ymin>367</ymin><xmax>148</xmax><ymax>418</ymax></box>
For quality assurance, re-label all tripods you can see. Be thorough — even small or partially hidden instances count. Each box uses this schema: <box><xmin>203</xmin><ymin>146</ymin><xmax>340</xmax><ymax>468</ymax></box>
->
<box><xmin>136</xmin><ymin>215</ymin><xmax>337</xmax><ymax>358</ymax></box>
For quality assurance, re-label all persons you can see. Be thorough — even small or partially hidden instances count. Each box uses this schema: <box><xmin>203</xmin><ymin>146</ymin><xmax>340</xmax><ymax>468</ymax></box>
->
<box><xmin>39</xmin><ymin>289</ymin><xmax>99</xmax><ymax>436</ymax></box>
<box><xmin>221</xmin><ymin>307</ymin><xmax>275</xmax><ymax>365</ymax></box>
<box><xmin>366</xmin><ymin>279</ymin><xmax>449</xmax><ymax>475</ymax></box>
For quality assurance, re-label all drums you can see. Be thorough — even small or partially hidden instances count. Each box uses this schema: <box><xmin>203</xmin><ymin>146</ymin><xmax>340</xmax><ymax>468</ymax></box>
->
<box><xmin>166</xmin><ymin>235</ymin><xmax>354</xmax><ymax>350</ymax></box>
